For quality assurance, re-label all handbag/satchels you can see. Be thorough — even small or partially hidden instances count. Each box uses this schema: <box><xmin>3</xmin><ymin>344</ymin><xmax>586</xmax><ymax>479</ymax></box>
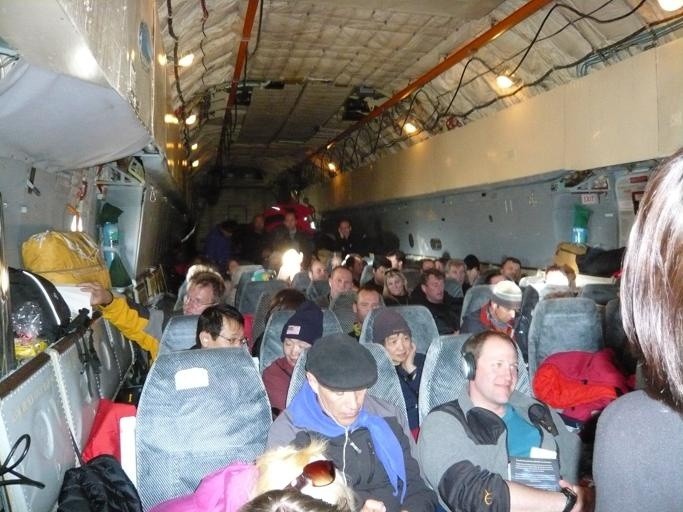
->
<box><xmin>554</xmin><ymin>240</ymin><xmax>626</xmax><ymax>278</ymax></box>
<box><xmin>22</xmin><ymin>230</ymin><xmax>112</xmax><ymax>298</ymax></box>
<box><xmin>8</xmin><ymin>266</ymin><xmax>70</xmax><ymax>345</ymax></box>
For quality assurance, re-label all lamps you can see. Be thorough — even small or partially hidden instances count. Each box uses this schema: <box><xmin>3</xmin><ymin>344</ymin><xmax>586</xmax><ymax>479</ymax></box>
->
<box><xmin>160</xmin><ymin>48</ymin><xmax>205</xmax><ymax>174</ymax></box>
<box><xmin>487</xmin><ymin>66</ymin><xmax>526</xmax><ymax>94</ymax></box>
<box><xmin>404</xmin><ymin>118</ymin><xmax>420</xmax><ymax>136</ymax></box>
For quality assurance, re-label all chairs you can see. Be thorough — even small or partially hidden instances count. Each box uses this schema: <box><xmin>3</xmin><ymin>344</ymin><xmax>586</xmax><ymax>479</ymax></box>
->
<box><xmin>0</xmin><ymin>242</ymin><xmax>683</xmax><ymax>510</ymax></box>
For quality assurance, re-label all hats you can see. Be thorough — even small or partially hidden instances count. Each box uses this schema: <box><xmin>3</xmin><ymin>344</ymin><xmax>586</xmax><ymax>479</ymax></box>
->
<box><xmin>490</xmin><ymin>280</ymin><xmax>522</xmax><ymax>309</ymax></box>
<box><xmin>305</xmin><ymin>335</ymin><xmax>377</xmax><ymax>389</ymax></box>
<box><xmin>281</xmin><ymin>301</ymin><xmax>322</xmax><ymax>346</ymax></box>
<box><xmin>374</xmin><ymin>311</ymin><xmax>411</xmax><ymax>344</ymax></box>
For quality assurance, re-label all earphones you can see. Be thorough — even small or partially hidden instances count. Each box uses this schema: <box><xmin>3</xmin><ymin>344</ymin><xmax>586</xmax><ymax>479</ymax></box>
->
<box><xmin>311</xmin><ymin>382</ymin><xmax>318</xmax><ymax>394</ymax></box>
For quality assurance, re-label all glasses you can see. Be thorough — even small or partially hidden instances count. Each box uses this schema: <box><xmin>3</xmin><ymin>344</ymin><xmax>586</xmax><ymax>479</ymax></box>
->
<box><xmin>214</xmin><ymin>331</ymin><xmax>249</xmax><ymax>343</ymax></box>
<box><xmin>284</xmin><ymin>460</ymin><xmax>337</xmax><ymax>496</ymax></box>
<box><xmin>182</xmin><ymin>295</ymin><xmax>216</xmax><ymax>307</ymax></box>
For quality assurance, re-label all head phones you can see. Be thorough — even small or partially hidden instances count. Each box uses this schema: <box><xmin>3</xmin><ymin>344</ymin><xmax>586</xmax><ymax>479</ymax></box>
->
<box><xmin>460</xmin><ymin>338</ymin><xmax>476</xmax><ymax>380</ymax></box>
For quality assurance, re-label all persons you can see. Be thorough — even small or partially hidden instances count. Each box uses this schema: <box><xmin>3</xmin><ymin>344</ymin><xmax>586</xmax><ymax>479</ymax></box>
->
<box><xmin>265</xmin><ymin>212</ymin><xmax>303</xmax><ymax>258</ymax></box>
<box><xmin>183</xmin><ymin>251</ymin><xmax>641</xmax><ymax>512</ymax></box>
<box><xmin>82</xmin><ymin>265</ymin><xmax>221</xmax><ymax>356</ymax></box>
<box><xmin>243</xmin><ymin>216</ymin><xmax>268</xmax><ymax>257</ymax></box>
<box><xmin>204</xmin><ymin>220</ymin><xmax>239</xmax><ymax>271</ymax></box>
<box><xmin>319</xmin><ymin>219</ymin><xmax>362</xmax><ymax>250</ymax></box>
<box><xmin>593</xmin><ymin>144</ymin><xmax>683</xmax><ymax>510</ymax></box>
